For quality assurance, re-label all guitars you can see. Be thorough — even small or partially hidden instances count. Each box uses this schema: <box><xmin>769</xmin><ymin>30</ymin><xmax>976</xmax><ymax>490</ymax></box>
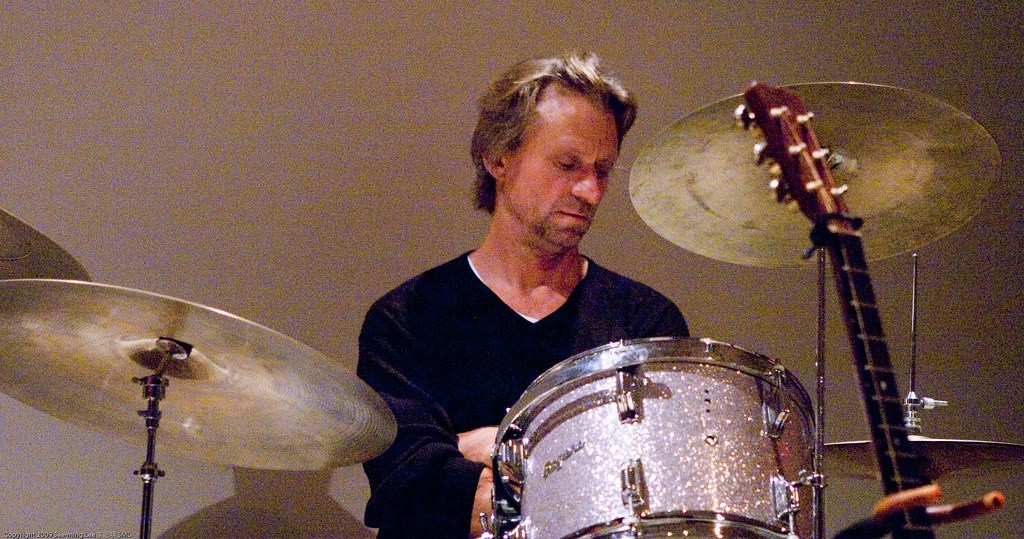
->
<box><xmin>736</xmin><ymin>81</ymin><xmax>1004</xmax><ymax>539</ymax></box>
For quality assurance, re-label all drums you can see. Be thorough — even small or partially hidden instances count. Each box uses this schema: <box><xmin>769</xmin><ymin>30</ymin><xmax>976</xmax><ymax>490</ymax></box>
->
<box><xmin>488</xmin><ymin>336</ymin><xmax>826</xmax><ymax>539</ymax></box>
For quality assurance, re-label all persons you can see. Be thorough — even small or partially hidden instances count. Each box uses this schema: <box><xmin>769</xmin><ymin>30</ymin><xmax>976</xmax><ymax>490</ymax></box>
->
<box><xmin>356</xmin><ymin>50</ymin><xmax>691</xmax><ymax>539</ymax></box>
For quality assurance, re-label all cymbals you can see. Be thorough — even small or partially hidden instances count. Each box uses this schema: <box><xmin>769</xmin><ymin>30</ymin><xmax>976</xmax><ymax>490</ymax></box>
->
<box><xmin>628</xmin><ymin>80</ymin><xmax>1002</xmax><ymax>270</ymax></box>
<box><xmin>821</xmin><ymin>436</ymin><xmax>1024</xmax><ymax>478</ymax></box>
<box><xmin>0</xmin><ymin>277</ymin><xmax>399</xmax><ymax>472</ymax></box>
<box><xmin>0</xmin><ymin>208</ymin><xmax>94</xmax><ymax>283</ymax></box>
